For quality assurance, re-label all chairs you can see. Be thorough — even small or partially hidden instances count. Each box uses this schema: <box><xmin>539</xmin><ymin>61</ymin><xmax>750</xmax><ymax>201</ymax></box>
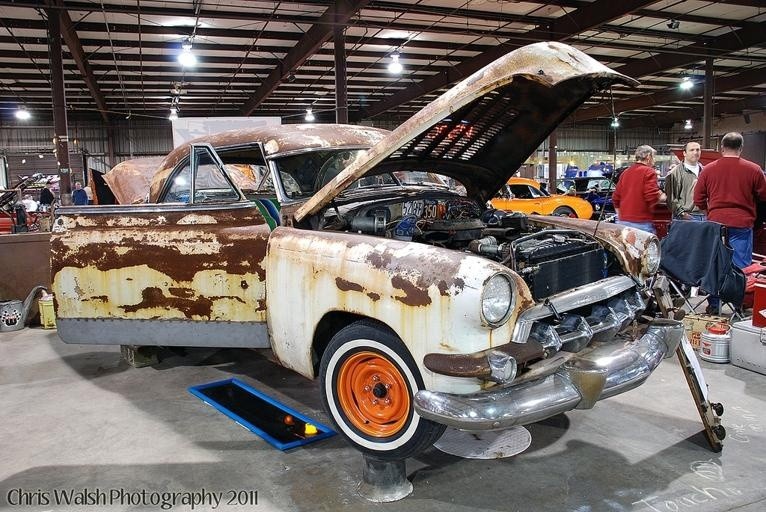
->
<box><xmin>666</xmin><ymin>220</ymin><xmax>766</xmax><ymax>321</ymax></box>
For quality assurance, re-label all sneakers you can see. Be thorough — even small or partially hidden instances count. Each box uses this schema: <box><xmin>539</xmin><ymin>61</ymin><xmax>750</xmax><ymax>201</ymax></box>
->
<box><xmin>671</xmin><ymin>289</ymin><xmax>719</xmax><ymax>315</ymax></box>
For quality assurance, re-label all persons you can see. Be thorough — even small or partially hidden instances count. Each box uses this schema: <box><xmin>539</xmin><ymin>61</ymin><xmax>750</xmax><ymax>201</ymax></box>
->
<box><xmin>693</xmin><ymin>131</ymin><xmax>766</xmax><ymax>316</ymax></box>
<box><xmin>72</xmin><ymin>182</ymin><xmax>89</xmax><ymax>205</ymax></box>
<box><xmin>84</xmin><ymin>184</ymin><xmax>93</xmax><ymax>205</ymax></box>
<box><xmin>611</xmin><ymin>145</ymin><xmax>667</xmax><ymax>235</ymax></box>
<box><xmin>39</xmin><ymin>180</ymin><xmax>56</xmax><ymax>205</ymax></box>
<box><xmin>21</xmin><ymin>194</ymin><xmax>38</xmax><ymax>212</ymax></box>
<box><xmin>664</xmin><ymin>142</ymin><xmax>706</xmax><ymax>298</ymax></box>
